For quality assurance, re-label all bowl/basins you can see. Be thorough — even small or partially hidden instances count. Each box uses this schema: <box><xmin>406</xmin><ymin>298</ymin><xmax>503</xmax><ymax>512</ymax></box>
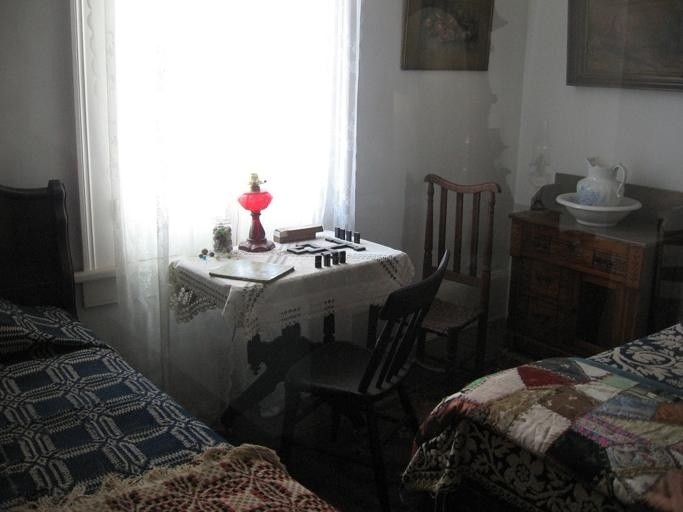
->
<box><xmin>555</xmin><ymin>193</ymin><xmax>642</xmax><ymax>229</ymax></box>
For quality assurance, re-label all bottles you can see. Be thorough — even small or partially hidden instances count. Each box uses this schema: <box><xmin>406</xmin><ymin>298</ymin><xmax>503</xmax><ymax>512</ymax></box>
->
<box><xmin>211</xmin><ymin>221</ymin><xmax>234</xmax><ymax>254</ymax></box>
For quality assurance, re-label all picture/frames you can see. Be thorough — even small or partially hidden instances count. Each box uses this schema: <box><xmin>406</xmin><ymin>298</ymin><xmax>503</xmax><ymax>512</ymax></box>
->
<box><xmin>566</xmin><ymin>2</ymin><xmax>682</xmax><ymax>90</ymax></box>
<box><xmin>401</xmin><ymin>0</ymin><xmax>493</xmax><ymax>71</ymax></box>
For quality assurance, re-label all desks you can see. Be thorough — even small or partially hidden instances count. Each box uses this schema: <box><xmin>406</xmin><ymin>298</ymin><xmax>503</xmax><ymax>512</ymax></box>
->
<box><xmin>168</xmin><ymin>230</ymin><xmax>416</xmax><ymax>430</ymax></box>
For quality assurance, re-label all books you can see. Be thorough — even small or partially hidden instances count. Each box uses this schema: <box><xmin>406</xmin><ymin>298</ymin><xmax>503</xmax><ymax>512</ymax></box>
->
<box><xmin>210</xmin><ymin>258</ymin><xmax>294</xmax><ymax>283</ymax></box>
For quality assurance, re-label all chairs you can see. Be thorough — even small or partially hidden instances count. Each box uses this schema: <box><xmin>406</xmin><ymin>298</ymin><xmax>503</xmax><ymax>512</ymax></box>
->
<box><xmin>397</xmin><ymin>172</ymin><xmax>499</xmax><ymax>388</ymax></box>
<box><xmin>278</xmin><ymin>252</ymin><xmax>457</xmax><ymax>471</ymax></box>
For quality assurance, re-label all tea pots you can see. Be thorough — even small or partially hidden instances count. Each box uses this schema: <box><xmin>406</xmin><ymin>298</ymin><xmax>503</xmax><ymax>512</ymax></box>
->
<box><xmin>577</xmin><ymin>155</ymin><xmax>629</xmax><ymax>205</ymax></box>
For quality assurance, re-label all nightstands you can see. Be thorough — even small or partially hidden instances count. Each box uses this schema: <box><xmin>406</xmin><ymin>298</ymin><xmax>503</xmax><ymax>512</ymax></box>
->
<box><xmin>505</xmin><ymin>208</ymin><xmax>656</xmax><ymax>362</ymax></box>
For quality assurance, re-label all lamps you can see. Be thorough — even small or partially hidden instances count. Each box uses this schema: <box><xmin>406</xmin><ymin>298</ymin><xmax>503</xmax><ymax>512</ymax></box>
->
<box><xmin>240</xmin><ymin>173</ymin><xmax>277</xmax><ymax>251</ymax></box>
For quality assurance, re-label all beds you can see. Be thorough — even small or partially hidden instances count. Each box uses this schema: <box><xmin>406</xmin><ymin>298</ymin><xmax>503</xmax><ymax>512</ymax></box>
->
<box><xmin>407</xmin><ymin>323</ymin><xmax>683</xmax><ymax>511</ymax></box>
<box><xmin>1</xmin><ymin>182</ymin><xmax>339</xmax><ymax>510</ymax></box>
